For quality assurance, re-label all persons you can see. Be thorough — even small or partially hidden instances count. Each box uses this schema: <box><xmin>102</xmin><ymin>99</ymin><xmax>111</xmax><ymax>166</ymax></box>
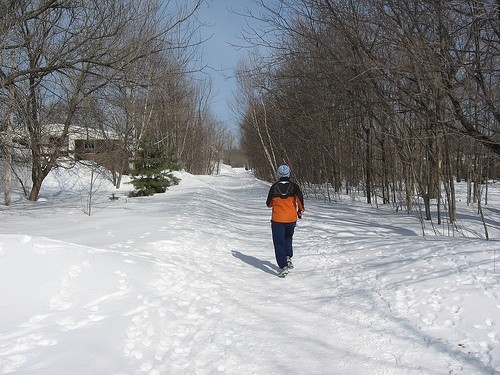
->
<box><xmin>266</xmin><ymin>165</ymin><xmax>305</xmax><ymax>277</ymax></box>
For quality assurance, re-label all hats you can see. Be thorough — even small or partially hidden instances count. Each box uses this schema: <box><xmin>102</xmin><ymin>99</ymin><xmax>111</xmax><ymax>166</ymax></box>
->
<box><xmin>276</xmin><ymin>164</ymin><xmax>291</xmax><ymax>179</ymax></box>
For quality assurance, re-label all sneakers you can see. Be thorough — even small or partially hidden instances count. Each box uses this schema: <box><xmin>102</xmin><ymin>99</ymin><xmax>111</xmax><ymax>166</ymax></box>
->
<box><xmin>277</xmin><ymin>266</ymin><xmax>289</xmax><ymax>278</ymax></box>
<box><xmin>286</xmin><ymin>259</ymin><xmax>294</xmax><ymax>269</ymax></box>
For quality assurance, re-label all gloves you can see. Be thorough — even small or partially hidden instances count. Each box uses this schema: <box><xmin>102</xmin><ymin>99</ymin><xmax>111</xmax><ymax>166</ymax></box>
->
<box><xmin>297</xmin><ymin>211</ymin><xmax>302</xmax><ymax>219</ymax></box>
